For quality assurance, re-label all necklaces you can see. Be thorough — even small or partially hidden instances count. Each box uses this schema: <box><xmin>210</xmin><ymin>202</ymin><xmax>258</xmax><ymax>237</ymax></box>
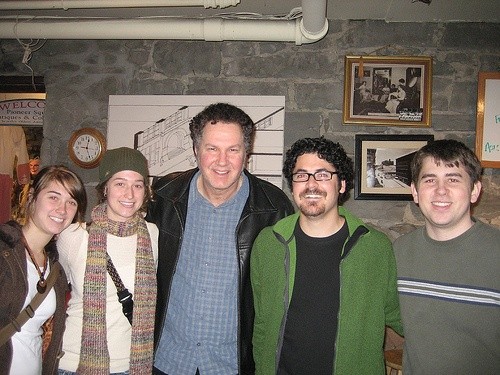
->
<box><xmin>24</xmin><ymin>240</ymin><xmax>48</xmax><ymax>294</ymax></box>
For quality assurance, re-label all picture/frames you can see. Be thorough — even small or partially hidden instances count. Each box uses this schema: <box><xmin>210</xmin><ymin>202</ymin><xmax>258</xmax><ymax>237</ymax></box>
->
<box><xmin>343</xmin><ymin>56</ymin><xmax>432</xmax><ymax>128</ymax></box>
<box><xmin>474</xmin><ymin>71</ymin><xmax>500</xmax><ymax>167</ymax></box>
<box><xmin>354</xmin><ymin>134</ymin><xmax>434</xmax><ymax>200</ymax></box>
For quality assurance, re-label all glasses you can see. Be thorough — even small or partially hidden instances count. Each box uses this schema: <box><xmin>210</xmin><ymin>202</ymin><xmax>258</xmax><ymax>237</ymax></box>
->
<box><xmin>290</xmin><ymin>171</ymin><xmax>339</xmax><ymax>182</ymax></box>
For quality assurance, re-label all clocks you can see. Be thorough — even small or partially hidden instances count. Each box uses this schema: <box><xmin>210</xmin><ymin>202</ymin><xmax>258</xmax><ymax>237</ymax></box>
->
<box><xmin>68</xmin><ymin>127</ymin><xmax>106</xmax><ymax>168</ymax></box>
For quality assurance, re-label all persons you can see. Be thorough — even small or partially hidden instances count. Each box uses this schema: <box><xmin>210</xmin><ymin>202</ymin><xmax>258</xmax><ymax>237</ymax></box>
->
<box><xmin>144</xmin><ymin>103</ymin><xmax>295</xmax><ymax>375</ymax></box>
<box><xmin>393</xmin><ymin>140</ymin><xmax>500</xmax><ymax>374</ymax></box>
<box><xmin>30</xmin><ymin>159</ymin><xmax>40</xmax><ymax>177</ymax></box>
<box><xmin>0</xmin><ymin>165</ymin><xmax>87</xmax><ymax>375</ymax></box>
<box><xmin>354</xmin><ymin>71</ymin><xmax>421</xmax><ymax>113</ymax></box>
<box><xmin>57</xmin><ymin>147</ymin><xmax>159</xmax><ymax>375</ymax></box>
<box><xmin>250</xmin><ymin>137</ymin><xmax>405</xmax><ymax>375</ymax></box>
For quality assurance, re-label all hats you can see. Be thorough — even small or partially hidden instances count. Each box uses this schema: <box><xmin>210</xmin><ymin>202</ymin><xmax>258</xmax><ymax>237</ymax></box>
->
<box><xmin>99</xmin><ymin>147</ymin><xmax>149</xmax><ymax>186</ymax></box>
